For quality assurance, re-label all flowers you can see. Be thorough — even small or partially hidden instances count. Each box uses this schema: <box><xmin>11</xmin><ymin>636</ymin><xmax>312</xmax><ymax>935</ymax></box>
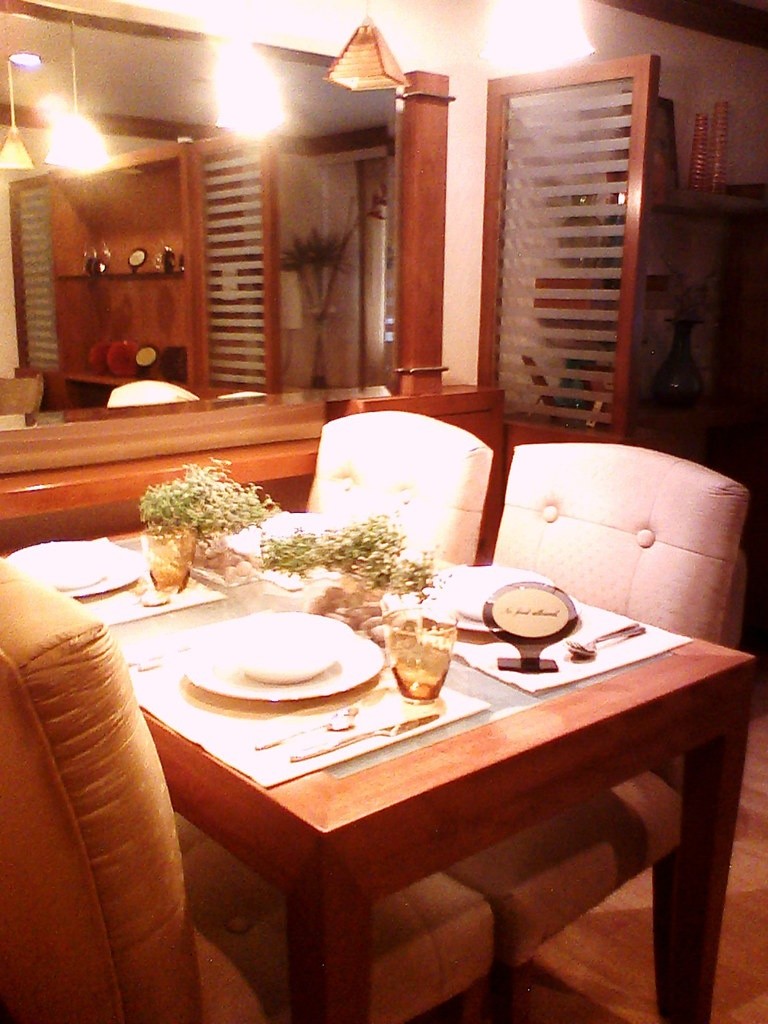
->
<box><xmin>273</xmin><ymin>190</ymin><xmax>385</xmax><ymax>310</ymax></box>
<box><xmin>138</xmin><ymin>456</ymin><xmax>280</xmax><ymax>538</ymax></box>
<box><xmin>257</xmin><ymin>510</ymin><xmax>439</xmax><ymax>605</ymax></box>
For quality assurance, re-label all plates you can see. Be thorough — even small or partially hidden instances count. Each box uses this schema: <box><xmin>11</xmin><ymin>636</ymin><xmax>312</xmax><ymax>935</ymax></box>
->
<box><xmin>418</xmin><ymin>565</ymin><xmax>581</xmax><ymax>633</ymax></box>
<box><xmin>180</xmin><ymin>632</ymin><xmax>385</xmax><ymax>701</ymax></box>
<box><xmin>7</xmin><ymin>543</ymin><xmax>141</xmax><ymax>600</ymax></box>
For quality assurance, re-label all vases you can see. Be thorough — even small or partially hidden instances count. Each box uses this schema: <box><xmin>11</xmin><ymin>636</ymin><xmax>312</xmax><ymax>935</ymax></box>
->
<box><xmin>190</xmin><ymin>535</ymin><xmax>259</xmax><ymax>587</ymax></box>
<box><xmin>555</xmin><ymin>358</ymin><xmax>589</xmax><ymax>410</ymax></box>
<box><xmin>305</xmin><ymin>311</ymin><xmax>331</xmax><ymax>387</ymax></box>
<box><xmin>558</xmin><ymin>170</ymin><xmax>608</xmax><ymax>274</ymax></box>
<box><xmin>651</xmin><ymin>314</ymin><xmax>706</xmax><ymax>412</ymax></box>
<box><xmin>311</xmin><ymin>580</ymin><xmax>391</xmax><ymax>647</ymax></box>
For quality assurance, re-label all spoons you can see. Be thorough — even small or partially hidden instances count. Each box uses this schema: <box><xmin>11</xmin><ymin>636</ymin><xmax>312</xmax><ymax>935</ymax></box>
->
<box><xmin>255</xmin><ymin>706</ymin><xmax>359</xmax><ymax>750</ymax></box>
<box><xmin>565</xmin><ymin>622</ymin><xmax>640</xmax><ymax>658</ymax></box>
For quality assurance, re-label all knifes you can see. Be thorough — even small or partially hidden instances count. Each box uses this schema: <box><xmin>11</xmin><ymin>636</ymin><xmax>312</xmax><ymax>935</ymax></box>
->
<box><xmin>290</xmin><ymin>714</ymin><xmax>442</xmax><ymax>763</ymax></box>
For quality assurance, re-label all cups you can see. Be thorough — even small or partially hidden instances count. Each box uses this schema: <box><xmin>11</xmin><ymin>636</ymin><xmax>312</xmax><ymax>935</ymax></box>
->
<box><xmin>382</xmin><ymin>610</ymin><xmax>460</xmax><ymax>708</ymax></box>
<box><xmin>141</xmin><ymin>528</ymin><xmax>198</xmax><ymax>594</ymax></box>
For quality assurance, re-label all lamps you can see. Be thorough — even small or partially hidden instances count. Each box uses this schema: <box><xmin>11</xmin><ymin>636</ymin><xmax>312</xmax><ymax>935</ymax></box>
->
<box><xmin>319</xmin><ymin>0</ymin><xmax>410</xmax><ymax>92</ymax></box>
<box><xmin>0</xmin><ymin>58</ymin><xmax>35</xmax><ymax>172</ymax></box>
<box><xmin>43</xmin><ymin>21</ymin><xmax>114</xmax><ymax>172</ymax></box>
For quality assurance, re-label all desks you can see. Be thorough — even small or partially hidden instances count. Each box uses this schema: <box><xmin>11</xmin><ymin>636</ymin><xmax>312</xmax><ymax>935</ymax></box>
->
<box><xmin>0</xmin><ymin>507</ymin><xmax>757</xmax><ymax>1024</ymax></box>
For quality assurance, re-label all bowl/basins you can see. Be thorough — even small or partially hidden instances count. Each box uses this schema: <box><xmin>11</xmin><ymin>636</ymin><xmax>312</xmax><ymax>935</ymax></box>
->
<box><xmin>229</xmin><ymin>612</ymin><xmax>356</xmax><ymax>684</ymax></box>
<box><xmin>42</xmin><ymin>541</ymin><xmax>110</xmax><ymax>591</ymax></box>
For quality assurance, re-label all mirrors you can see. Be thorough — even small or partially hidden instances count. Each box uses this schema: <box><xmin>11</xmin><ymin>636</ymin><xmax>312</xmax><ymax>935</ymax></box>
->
<box><xmin>0</xmin><ymin>0</ymin><xmax>405</xmax><ymax>429</ymax></box>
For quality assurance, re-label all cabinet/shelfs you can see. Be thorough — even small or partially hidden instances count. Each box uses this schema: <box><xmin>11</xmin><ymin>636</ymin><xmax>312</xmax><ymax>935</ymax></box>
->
<box><xmin>51</xmin><ymin>142</ymin><xmax>204</xmax><ymax>386</ymax></box>
<box><xmin>504</xmin><ymin>189</ymin><xmax>760</xmax><ymax>433</ymax></box>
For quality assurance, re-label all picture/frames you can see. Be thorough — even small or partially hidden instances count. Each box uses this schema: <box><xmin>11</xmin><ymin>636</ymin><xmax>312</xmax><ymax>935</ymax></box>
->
<box><xmin>521</xmin><ymin>354</ymin><xmax>562</xmax><ymax>424</ymax></box>
<box><xmin>604</xmin><ymin>88</ymin><xmax>684</xmax><ymax>190</ymax></box>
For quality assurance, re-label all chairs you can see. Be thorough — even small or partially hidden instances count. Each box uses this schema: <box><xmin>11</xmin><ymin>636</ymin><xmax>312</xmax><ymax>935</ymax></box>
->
<box><xmin>0</xmin><ymin>411</ymin><xmax>754</xmax><ymax>1024</ymax></box>
<box><xmin>0</xmin><ymin>373</ymin><xmax>45</xmax><ymax>430</ymax></box>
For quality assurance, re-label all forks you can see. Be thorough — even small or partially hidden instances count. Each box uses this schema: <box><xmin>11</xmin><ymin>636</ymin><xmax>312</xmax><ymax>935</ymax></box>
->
<box><xmin>566</xmin><ymin>627</ymin><xmax>646</xmax><ymax>652</ymax></box>
